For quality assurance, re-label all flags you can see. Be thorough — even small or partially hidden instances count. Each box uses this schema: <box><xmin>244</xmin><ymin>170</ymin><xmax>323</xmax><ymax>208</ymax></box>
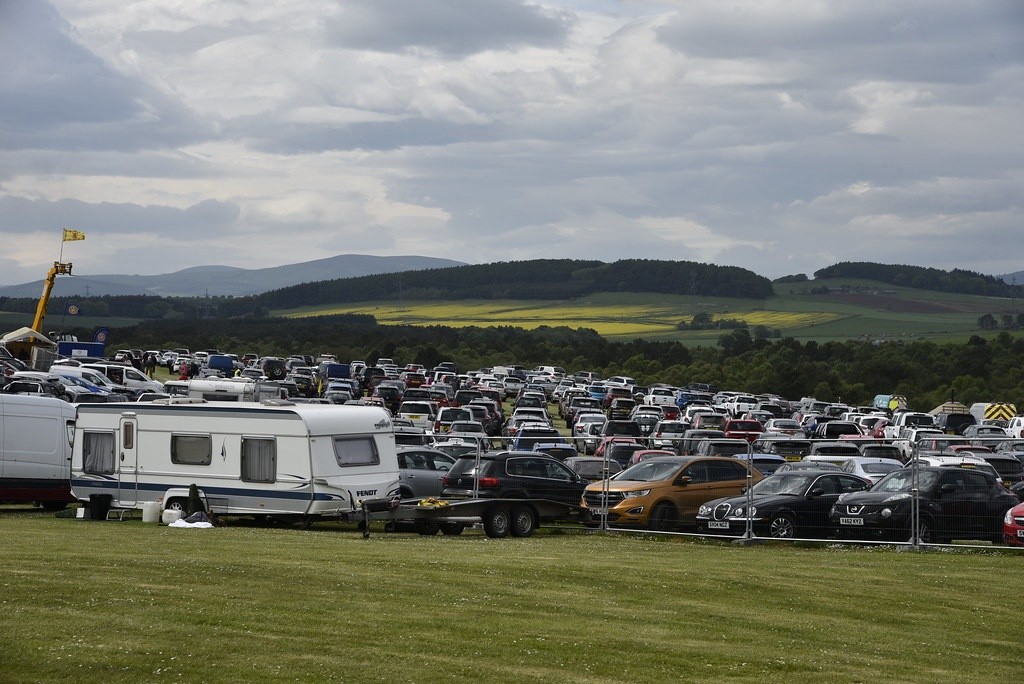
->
<box><xmin>64</xmin><ymin>229</ymin><xmax>85</xmax><ymax>242</ymax></box>
<box><xmin>65</xmin><ymin>304</ymin><xmax>81</xmax><ymax>318</ymax></box>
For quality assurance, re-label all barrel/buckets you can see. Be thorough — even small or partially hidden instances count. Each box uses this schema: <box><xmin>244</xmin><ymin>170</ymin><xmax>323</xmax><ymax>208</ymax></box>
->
<box><xmin>89</xmin><ymin>494</ymin><xmax>112</xmax><ymax>520</ymax></box>
<box><xmin>142</xmin><ymin>501</ymin><xmax>160</xmax><ymax>523</ymax></box>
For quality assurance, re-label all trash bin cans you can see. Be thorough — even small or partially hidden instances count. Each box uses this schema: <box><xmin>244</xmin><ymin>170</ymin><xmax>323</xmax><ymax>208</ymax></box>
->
<box><xmin>89</xmin><ymin>493</ymin><xmax>112</xmax><ymax>520</ymax></box>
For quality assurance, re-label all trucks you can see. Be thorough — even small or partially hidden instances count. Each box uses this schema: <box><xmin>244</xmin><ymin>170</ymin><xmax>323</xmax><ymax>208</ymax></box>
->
<box><xmin>65</xmin><ymin>398</ymin><xmax>402</xmax><ymax>527</ymax></box>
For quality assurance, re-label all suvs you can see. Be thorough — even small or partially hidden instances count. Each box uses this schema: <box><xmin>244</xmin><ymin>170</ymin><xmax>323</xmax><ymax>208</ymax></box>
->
<box><xmin>439</xmin><ymin>449</ymin><xmax>594</xmax><ymax>539</ymax></box>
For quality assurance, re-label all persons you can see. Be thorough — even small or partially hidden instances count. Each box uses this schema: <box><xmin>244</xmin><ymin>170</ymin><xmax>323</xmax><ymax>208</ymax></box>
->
<box><xmin>123</xmin><ymin>351</ymin><xmax>156</xmax><ymax>379</ymax></box>
<box><xmin>189</xmin><ymin>362</ymin><xmax>199</xmax><ymax>379</ymax></box>
<box><xmin>167</xmin><ymin>356</ymin><xmax>175</xmax><ymax>375</ymax></box>
<box><xmin>181</xmin><ymin>362</ymin><xmax>187</xmax><ymax>375</ymax></box>
<box><xmin>234</xmin><ymin>366</ymin><xmax>242</xmax><ymax>377</ymax></box>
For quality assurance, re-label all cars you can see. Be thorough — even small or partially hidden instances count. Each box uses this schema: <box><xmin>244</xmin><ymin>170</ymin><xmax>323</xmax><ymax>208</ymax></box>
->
<box><xmin>579</xmin><ymin>455</ymin><xmax>767</xmax><ymax>536</ymax></box>
<box><xmin>1004</xmin><ymin>500</ymin><xmax>1024</xmax><ymax>550</ymax></box>
<box><xmin>0</xmin><ymin>344</ymin><xmax>1024</xmax><ymax>468</ymax></box>
<box><xmin>827</xmin><ymin>464</ymin><xmax>1021</xmax><ymax>548</ymax></box>
<box><xmin>395</xmin><ymin>446</ymin><xmax>458</xmax><ymax>506</ymax></box>
<box><xmin>695</xmin><ymin>469</ymin><xmax>875</xmax><ymax>544</ymax></box>
